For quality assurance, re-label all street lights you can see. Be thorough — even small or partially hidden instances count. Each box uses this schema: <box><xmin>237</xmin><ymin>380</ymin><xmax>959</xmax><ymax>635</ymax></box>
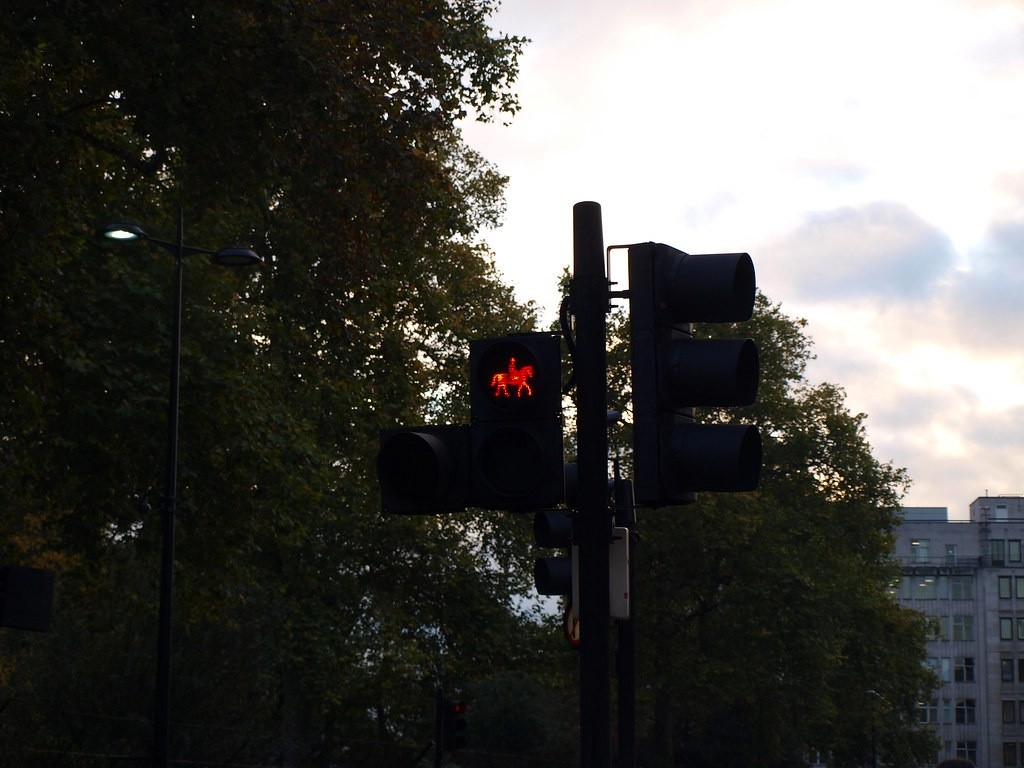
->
<box><xmin>102</xmin><ymin>207</ymin><xmax>261</xmax><ymax>768</ymax></box>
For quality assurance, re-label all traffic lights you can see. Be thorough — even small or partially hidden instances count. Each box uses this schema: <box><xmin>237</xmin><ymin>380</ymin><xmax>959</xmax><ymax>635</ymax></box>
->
<box><xmin>468</xmin><ymin>334</ymin><xmax>562</xmax><ymax>509</ymax></box>
<box><xmin>376</xmin><ymin>424</ymin><xmax>468</xmax><ymax>514</ymax></box>
<box><xmin>443</xmin><ymin>696</ymin><xmax>468</xmax><ymax>753</ymax></box>
<box><xmin>533</xmin><ymin>460</ymin><xmax>579</xmax><ymax>618</ymax></box>
<box><xmin>627</xmin><ymin>242</ymin><xmax>764</xmax><ymax>502</ymax></box>
